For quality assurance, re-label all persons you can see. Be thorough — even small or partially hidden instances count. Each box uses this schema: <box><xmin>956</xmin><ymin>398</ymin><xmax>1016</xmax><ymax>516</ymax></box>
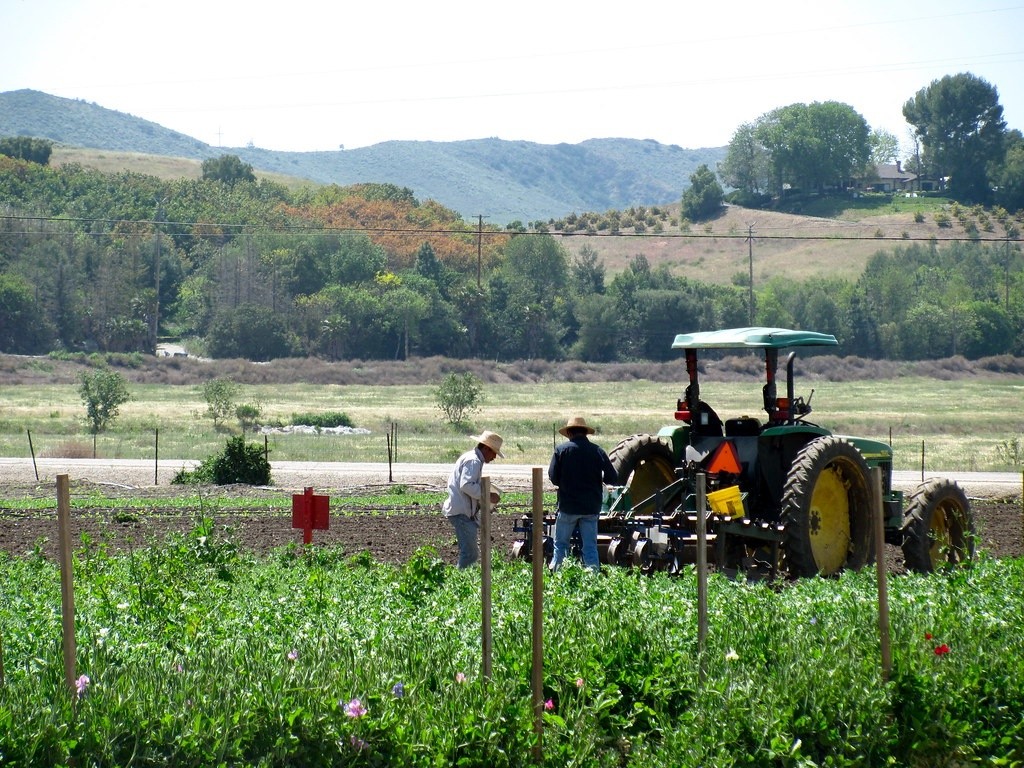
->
<box><xmin>548</xmin><ymin>417</ymin><xmax>618</xmax><ymax>575</ymax></box>
<box><xmin>443</xmin><ymin>431</ymin><xmax>504</xmax><ymax>569</ymax></box>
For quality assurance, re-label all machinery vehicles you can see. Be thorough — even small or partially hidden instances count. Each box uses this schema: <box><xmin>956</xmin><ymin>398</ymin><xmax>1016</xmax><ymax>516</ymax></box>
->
<box><xmin>511</xmin><ymin>328</ymin><xmax>973</xmax><ymax>579</ymax></box>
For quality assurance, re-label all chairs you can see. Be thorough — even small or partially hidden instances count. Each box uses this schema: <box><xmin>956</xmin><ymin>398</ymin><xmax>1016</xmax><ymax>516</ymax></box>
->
<box><xmin>726</xmin><ymin>418</ymin><xmax>764</xmax><ymax>436</ymax></box>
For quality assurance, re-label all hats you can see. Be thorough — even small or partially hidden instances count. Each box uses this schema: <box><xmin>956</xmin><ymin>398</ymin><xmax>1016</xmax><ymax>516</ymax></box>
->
<box><xmin>559</xmin><ymin>416</ymin><xmax>595</xmax><ymax>438</ymax></box>
<box><xmin>469</xmin><ymin>430</ymin><xmax>506</xmax><ymax>459</ymax></box>
